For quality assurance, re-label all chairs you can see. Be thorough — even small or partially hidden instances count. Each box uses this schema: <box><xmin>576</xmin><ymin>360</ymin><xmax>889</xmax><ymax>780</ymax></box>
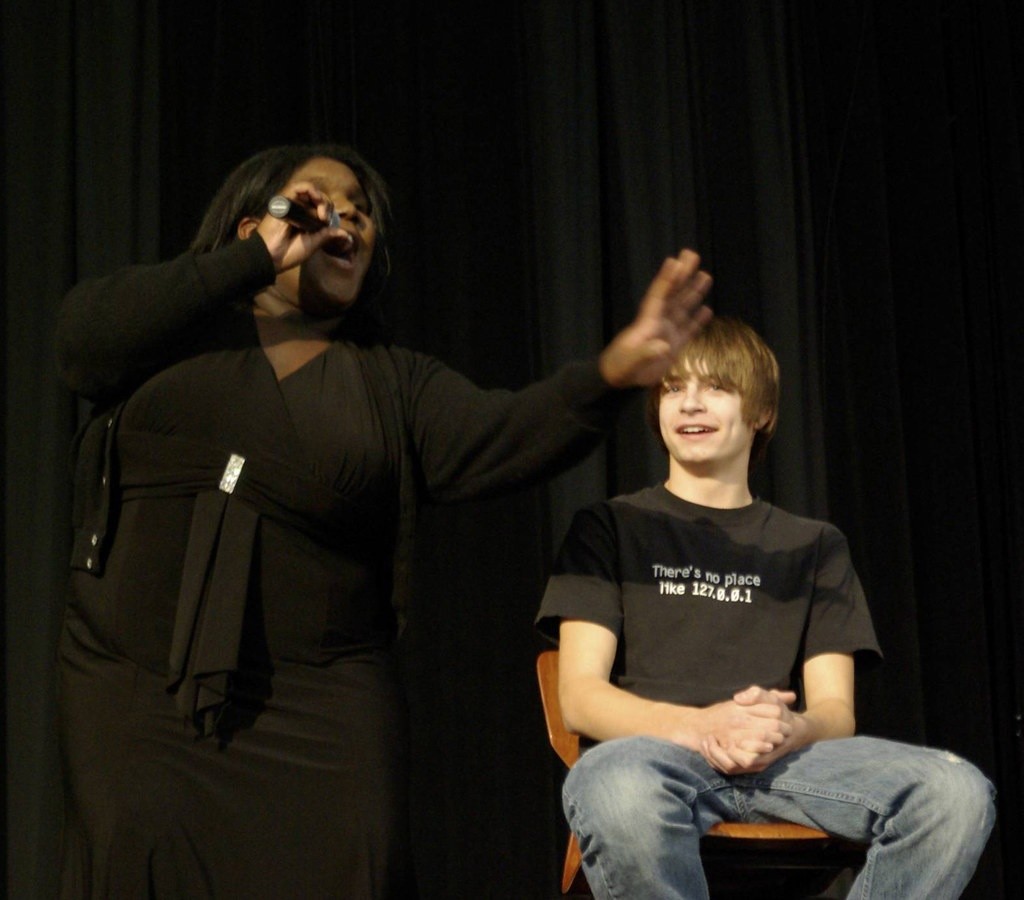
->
<box><xmin>537</xmin><ymin>650</ymin><xmax>866</xmax><ymax>900</ymax></box>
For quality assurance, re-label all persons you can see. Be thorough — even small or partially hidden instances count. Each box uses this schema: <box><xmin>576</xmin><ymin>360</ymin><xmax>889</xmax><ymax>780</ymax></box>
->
<box><xmin>31</xmin><ymin>147</ymin><xmax>713</xmax><ymax>900</ymax></box>
<box><xmin>533</xmin><ymin>315</ymin><xmax>996</xmax><ymax>900</ymax></box>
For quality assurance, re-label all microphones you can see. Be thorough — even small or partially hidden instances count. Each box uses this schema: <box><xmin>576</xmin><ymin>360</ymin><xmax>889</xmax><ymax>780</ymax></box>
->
<box><xmin>267</xmin><ymin>195</ymin><xmax>355</xmax><ymax>256</ymax></box>
